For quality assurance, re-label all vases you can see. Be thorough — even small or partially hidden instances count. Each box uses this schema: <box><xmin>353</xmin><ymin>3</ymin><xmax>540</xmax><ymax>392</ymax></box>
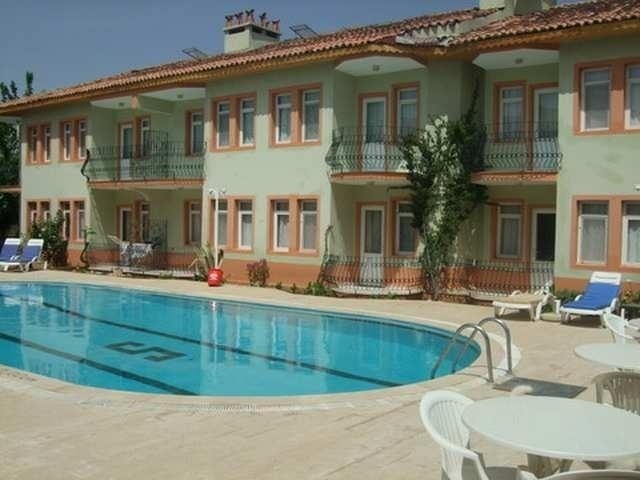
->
<box><xmin>251</xmin><ymin>277</ymin><xmax>265</xmax><ymax>286</ymax></box>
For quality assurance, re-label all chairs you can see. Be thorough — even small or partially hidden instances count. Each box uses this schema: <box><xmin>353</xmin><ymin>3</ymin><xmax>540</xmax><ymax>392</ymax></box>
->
<box><xmin>0</xmin><ymin>236</ymin><xmax>49</xmax><ymax>275</ymax></box>
<box><xmin>492</xmin><ymin>270</ymin><xmax>639</xmax><ymax>339</ymax></box>
<box><xmin>418</xmin><ymin>370</ymin><xmax>639</xmax><ymax>480</ymax></box>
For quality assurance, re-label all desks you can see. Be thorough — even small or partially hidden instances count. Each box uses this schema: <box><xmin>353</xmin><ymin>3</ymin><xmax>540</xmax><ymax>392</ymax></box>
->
<box><xmin>575</xmin><ymin>343</ymin><xmax>640</xmax><ymax>371</ymax></box>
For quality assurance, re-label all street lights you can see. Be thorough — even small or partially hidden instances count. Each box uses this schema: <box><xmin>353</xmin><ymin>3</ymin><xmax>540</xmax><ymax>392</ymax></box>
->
<box><xmin>208</xmin><ymin>188</ymin><xmax>227</xmax><ymax>268</ymax></box>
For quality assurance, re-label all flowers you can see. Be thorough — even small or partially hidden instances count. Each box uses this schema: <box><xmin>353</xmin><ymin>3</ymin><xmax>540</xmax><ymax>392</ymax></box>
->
<box><xmin>244</xmin><ymin>257</ymin><xmax>270</xmax><ymax>279</ymax></box>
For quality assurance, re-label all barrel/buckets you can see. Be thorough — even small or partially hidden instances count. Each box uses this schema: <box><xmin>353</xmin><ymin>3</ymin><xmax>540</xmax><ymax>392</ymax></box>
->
<box><xmin>208</xmin><ymin>268</ymin><xmax>223</xmax><ymax>286</ymax></box>
